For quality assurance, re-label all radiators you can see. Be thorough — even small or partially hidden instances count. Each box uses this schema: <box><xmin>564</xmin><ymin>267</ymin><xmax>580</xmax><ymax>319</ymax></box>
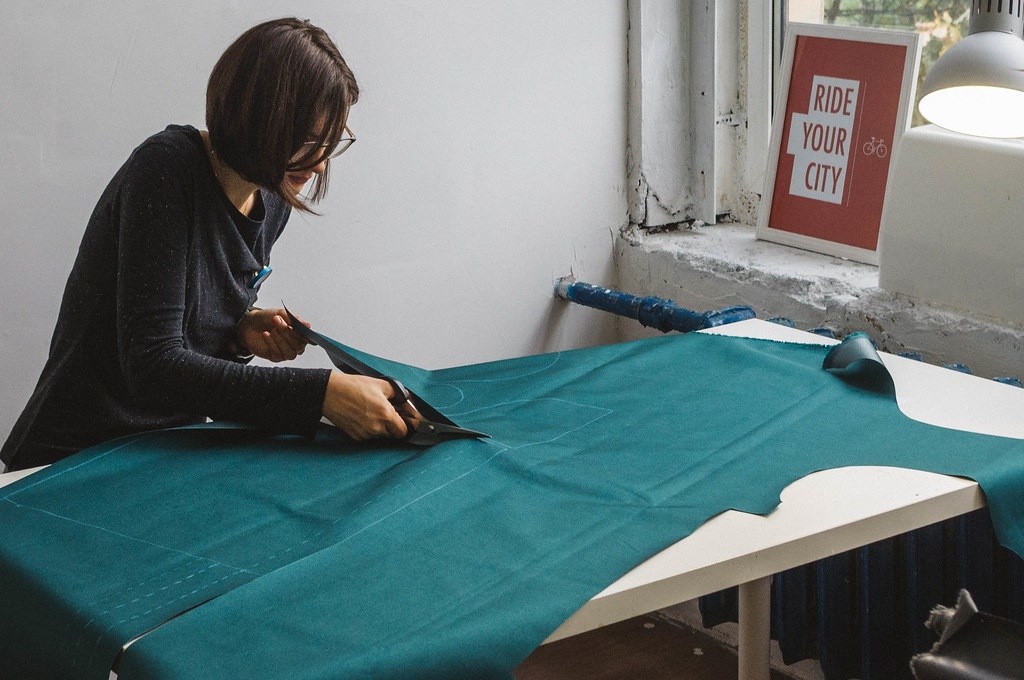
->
<box><xmin>697</xmin><ymin>305</ymin><xmax>1023</xmax><ymax>680</ymax></box>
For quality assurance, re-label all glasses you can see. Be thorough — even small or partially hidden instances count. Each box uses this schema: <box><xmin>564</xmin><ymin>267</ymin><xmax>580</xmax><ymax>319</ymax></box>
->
<box><xmin>289</xmin><ymin>123</ymin><xmax>357</xmax><ymax>164</ymax></box>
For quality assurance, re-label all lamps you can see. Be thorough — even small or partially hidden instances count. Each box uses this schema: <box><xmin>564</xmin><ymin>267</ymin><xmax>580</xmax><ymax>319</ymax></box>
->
<box><xmin>917</xmin><ymin>0</ymin><xmax>1024</xmax><ymax>139</ymax></box>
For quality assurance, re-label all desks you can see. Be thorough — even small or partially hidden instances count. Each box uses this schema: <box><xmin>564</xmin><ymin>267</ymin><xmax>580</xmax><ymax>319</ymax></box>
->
<box><xmin>0</xmin><ymin>318</ymin><xmax>1024</xmax><ymax>680</ymax></box>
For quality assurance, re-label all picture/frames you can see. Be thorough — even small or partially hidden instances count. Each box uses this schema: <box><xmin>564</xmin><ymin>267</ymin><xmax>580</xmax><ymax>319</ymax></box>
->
<box><xmin>753</xmin><ymin>21</ymin><xmax>921</xmax><ymax>266</ymax></box>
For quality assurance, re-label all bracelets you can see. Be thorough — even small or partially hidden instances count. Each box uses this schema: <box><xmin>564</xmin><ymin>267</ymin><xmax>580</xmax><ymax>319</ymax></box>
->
<box><xmin>231</xmin><ymin>307</ymin><xmax>263</xmax><ymax>359</ymax></box>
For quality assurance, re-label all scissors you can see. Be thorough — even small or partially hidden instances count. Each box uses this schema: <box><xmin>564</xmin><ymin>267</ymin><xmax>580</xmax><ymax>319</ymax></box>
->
<box><xmin>376</xmin><ymin>375</ymin><xmax>493</xmax><ymax>446</ymax></box>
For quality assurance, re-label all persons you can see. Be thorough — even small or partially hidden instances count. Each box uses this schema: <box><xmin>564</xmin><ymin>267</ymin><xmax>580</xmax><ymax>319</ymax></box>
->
<box><xmin>0</xmin><ymin>17</ymin><xmax>413</xmax><ymax>474</ymax></box>
